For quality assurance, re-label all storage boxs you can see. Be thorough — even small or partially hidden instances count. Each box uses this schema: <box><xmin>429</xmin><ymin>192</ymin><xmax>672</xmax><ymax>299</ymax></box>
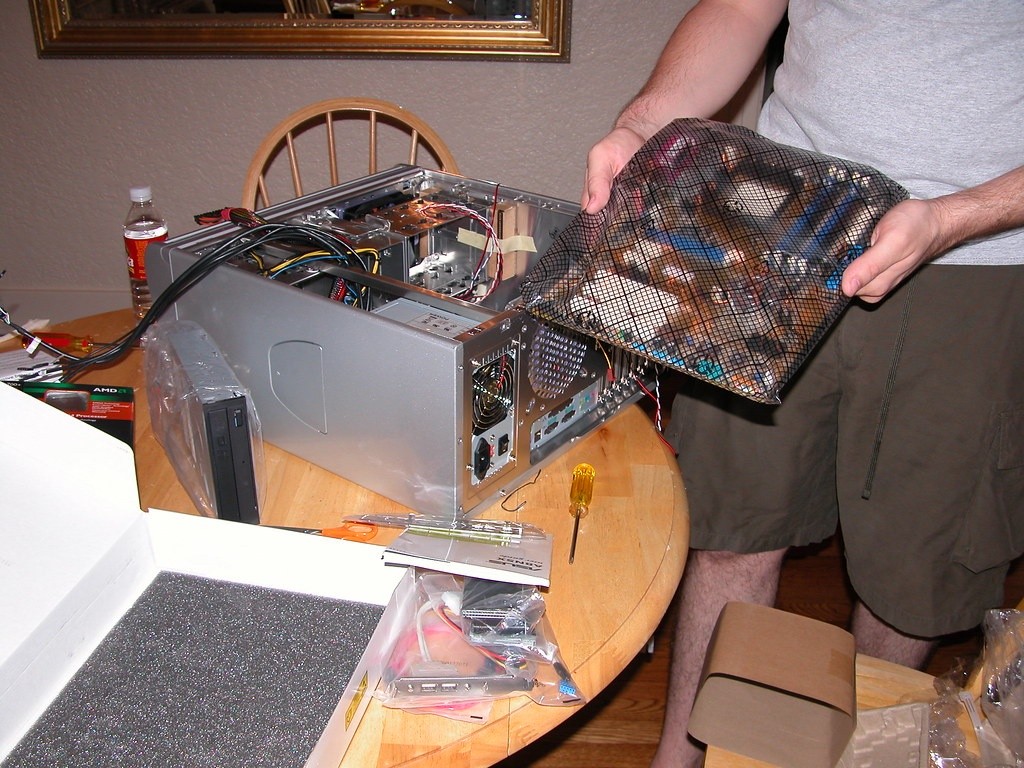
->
<box><xmin>686</xmin><ymin>599</ymin><xmax>858</xmax><ymax>767</ymax></box>
<box><xmin>0</xmin><ymin>381</ymin><xmax>415</xmax><ymax>768</ymax></box>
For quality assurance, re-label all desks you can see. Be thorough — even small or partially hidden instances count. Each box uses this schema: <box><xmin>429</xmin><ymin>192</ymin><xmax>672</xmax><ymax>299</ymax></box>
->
<box><xmin>0</xmin><ymin>306</ymin><xmax>691</xmax><ymax>768</ymax></box>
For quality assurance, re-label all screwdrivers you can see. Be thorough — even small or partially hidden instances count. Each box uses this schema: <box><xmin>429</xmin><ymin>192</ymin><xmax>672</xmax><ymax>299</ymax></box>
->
<box><xmin>568</xmin><ymin>462</ymin><xmax>596</xmax><ymax>566</ymax></box>
<box><xmin>21</xmin><ymin>331</ymin><xmax>146</xmax><ymax>355</ymax></box>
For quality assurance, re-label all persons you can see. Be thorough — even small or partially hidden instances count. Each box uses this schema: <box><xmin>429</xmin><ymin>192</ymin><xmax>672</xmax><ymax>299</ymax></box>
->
<box><xmin>583</xmin><ymin>0</ymin><xmax>1024</xmax><ymax>768</ymax></box>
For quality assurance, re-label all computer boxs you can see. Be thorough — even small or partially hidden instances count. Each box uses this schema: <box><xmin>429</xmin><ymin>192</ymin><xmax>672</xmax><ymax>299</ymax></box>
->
<box><xmin>143</xmin><ymin>163</ymin><xmax>665</xmax><ymax>521</ymax></box>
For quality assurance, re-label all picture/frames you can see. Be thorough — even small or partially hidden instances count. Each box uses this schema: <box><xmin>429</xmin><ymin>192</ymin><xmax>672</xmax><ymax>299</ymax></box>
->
<box><xmin>30</xmin><ymin>0</ymin><xmax>574</xmax><ymax>64</ymax></box>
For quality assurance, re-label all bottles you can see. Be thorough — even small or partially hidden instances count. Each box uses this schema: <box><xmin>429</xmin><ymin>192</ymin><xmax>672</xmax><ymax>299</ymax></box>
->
<box><xmin>123</xmin><ymin>185</ymin><xmax>168</xmax><ymax>320</ymax></box>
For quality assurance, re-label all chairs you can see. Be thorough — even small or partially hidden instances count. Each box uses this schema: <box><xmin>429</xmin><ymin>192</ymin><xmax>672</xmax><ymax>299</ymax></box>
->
<box><xmin>241</xmin><ymin>96</ymin><xmax>461</xmax><ymax>211</ymax></box>
<box><xmin>705</xmin><ymin>653</ymin><xmax>1024</xmax><ymax>768</ymax></box>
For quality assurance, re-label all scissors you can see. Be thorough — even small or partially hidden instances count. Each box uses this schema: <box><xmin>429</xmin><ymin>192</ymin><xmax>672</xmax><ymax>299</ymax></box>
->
<box><xmin>262</xmin><ymin>520</ymin><xmax>379</xmax><ymax>543</ymax></box>
